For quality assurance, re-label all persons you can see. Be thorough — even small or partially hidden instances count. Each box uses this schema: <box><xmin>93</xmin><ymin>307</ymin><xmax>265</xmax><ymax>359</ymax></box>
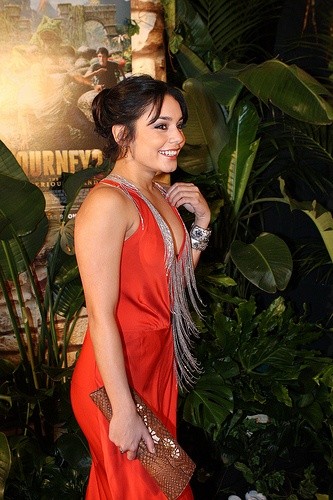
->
<box><xmin>63</xmin><ymin>43</ymin><xmax>127</xmax><ymax>143</ymax></box>
<box><xmin>69</xmin><ymin>75</ymin><xmax>212</xmax><ymax>500</ymax></box>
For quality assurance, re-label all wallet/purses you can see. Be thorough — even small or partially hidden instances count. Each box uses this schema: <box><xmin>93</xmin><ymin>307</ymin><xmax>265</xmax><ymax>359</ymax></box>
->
<box><xmin>89</xmin><ymin>378</ymin><xmax>200</xmax><ymax>500</ymax></box>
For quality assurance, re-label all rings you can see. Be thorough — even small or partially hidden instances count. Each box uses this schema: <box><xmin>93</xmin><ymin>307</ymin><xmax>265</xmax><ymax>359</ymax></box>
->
<box><xmin>119</xmin><ymin>447</ymin><xmax>124</xmax><ymax>454</ymax></box>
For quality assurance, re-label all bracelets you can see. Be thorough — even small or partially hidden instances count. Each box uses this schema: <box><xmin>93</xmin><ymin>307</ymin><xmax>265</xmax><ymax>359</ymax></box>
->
<box><xmin>189</xmin><ymin>221</ymin><xmax>212</xmax><ymax>251</ymax></box>
